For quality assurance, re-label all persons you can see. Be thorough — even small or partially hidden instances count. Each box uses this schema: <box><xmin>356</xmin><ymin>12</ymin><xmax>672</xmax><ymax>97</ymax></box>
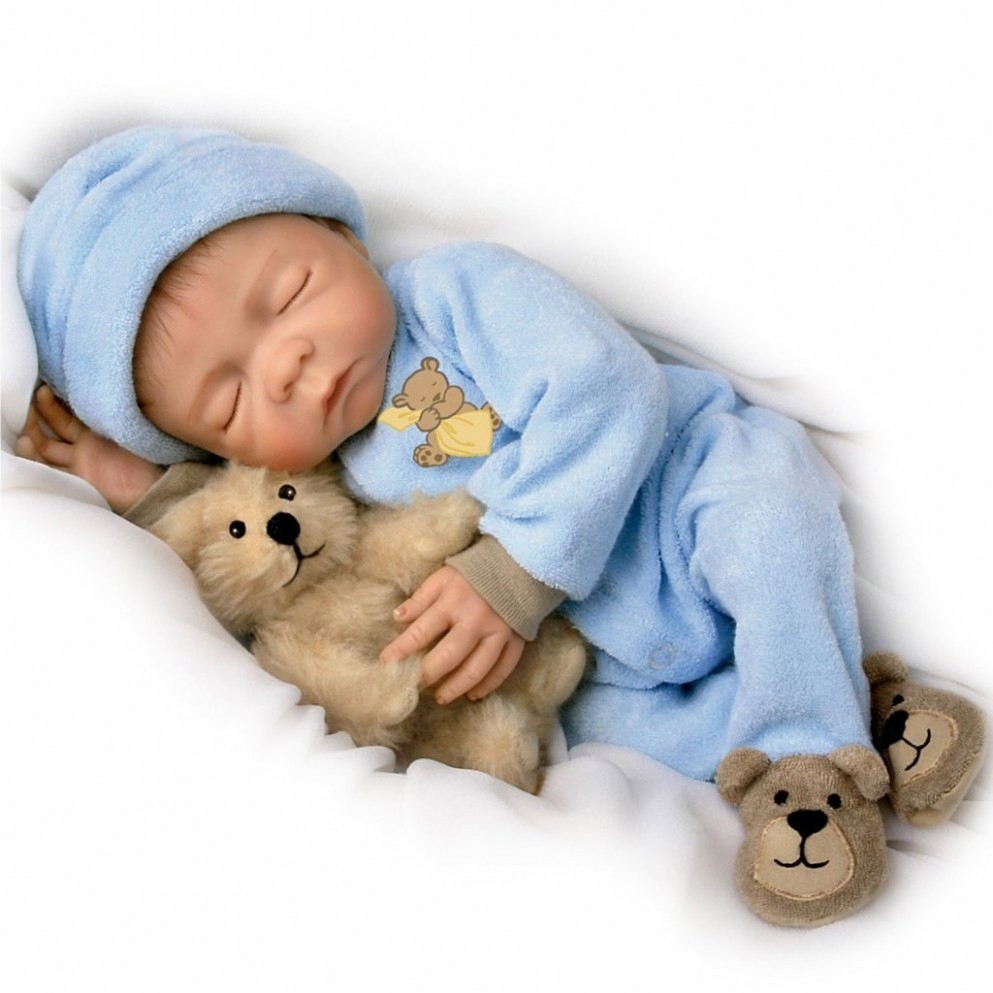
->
<box><xmin>11</xmin><ymin>124</ymin><xmax>988</xmax><ymax>930</ymax></box>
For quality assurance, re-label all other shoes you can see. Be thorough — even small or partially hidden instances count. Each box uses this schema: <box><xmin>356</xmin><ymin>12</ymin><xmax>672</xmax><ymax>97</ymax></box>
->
<box><xmin>717</xmin><ymin>745</ymin><xmax>891</xmax><ymax>929</ymax></box>
<box><xmin>859</xmin><ymin>651</ymin><xmax>992</xmax><ymax>828</ymax></box>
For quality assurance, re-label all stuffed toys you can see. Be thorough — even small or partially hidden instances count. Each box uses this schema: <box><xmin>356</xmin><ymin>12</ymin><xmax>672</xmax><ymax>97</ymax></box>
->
<box><xmin>145</xmin><ymin>459</ymin><xmax>589</xmax><ymax>796</ymax></box>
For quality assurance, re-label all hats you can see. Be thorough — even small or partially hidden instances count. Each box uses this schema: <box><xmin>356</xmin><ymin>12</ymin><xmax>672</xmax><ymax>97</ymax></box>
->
<box><xmin>16</xmin><ymin>121</ymin><xmax>369</xmax><ymax>466</ymax></box>
